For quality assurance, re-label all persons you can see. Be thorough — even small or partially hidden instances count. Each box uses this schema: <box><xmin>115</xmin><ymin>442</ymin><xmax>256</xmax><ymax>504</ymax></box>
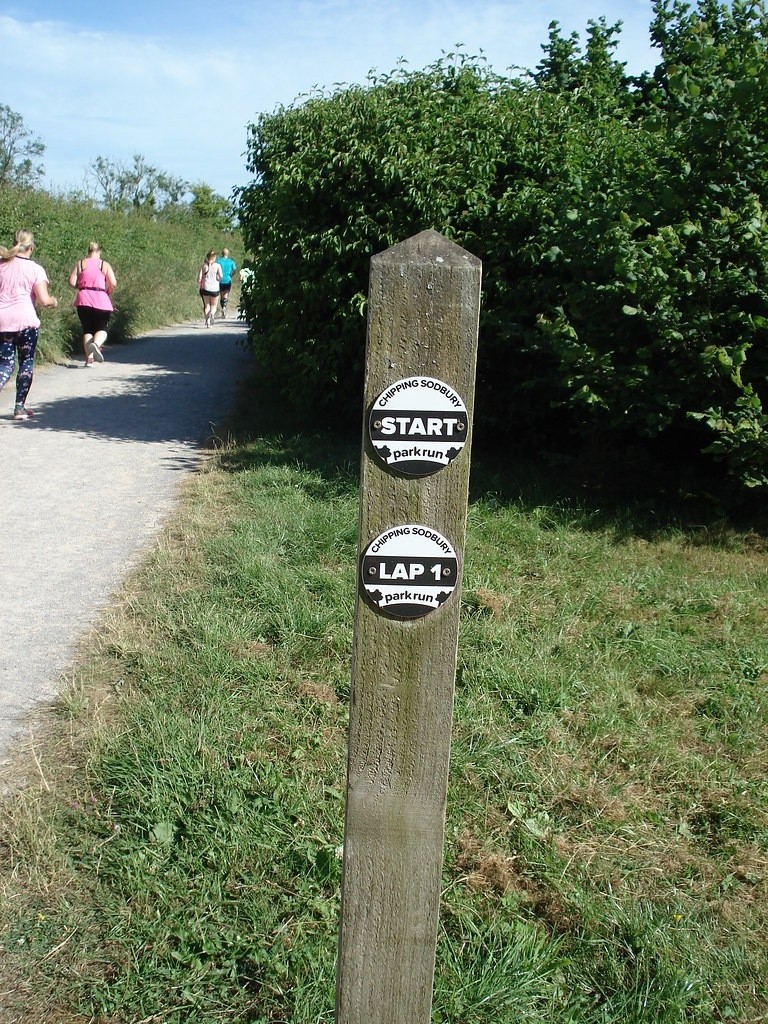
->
<box><xmin>68</xmin><ymin>241</ymin><xmax>117</xmax><ymax>366</ymax></box>
<box><xmin>0</xmin><ymin>229</ymin><xmax>58</xmax><ymax>419</ymax></box>
<box><xmin>197</xmin><ymin>247</ymin><xmax>257</xmax><ymax>327</ymax></box>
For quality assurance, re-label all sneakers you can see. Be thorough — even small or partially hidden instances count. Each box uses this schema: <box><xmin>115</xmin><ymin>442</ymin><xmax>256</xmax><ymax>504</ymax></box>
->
<box><xmin>89</xmin><ymin>342</ymin><xmax>104</xmax><ymax>363</ymax></box>
<box><xmin>86</xmin><ymin>357</ymin><xmax>94</xmax><ymax>366</ymax></box>
<box><xmin>14</xmin><ymin>404</ymin><xmax>33</xmax><ymax>419</ymax></box>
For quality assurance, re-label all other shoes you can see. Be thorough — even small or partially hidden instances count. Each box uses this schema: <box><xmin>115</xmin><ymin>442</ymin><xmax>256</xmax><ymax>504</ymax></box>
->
<box><xmin>207</xmin><ymin>322</ymin><xmax>212</xmax><ymax>328</ymax></box>
<box><xmin>221</xmin><ymin>309</ymin><xmax>225</xmax><ymax>316</ymax></box>
<box><xmin>209</xmin><ymin>312</ymin><xmax>215</xmax><ymax>325</ymax></box>
<box><xmin>222</xmin><ymin>315</ymin><xmax>228</xmax><ymax>318</ymax></box>
